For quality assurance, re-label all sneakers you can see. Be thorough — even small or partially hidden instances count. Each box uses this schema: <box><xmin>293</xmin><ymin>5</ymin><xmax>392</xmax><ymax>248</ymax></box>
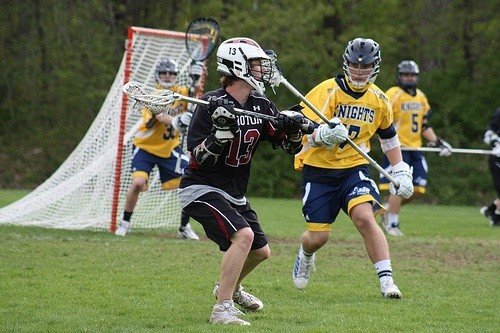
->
<box><xmin>115</xmin><ymin>219</ymin><xmax>130</xmax><ymax>236</ymax></box>
<box><xmin>210</xmin><ymin>304</ymin><xmax>252</xmax><ymax>327</ymax></box>
<box><xmin>379</xmin><ymin>272</ymin><xmax>403</xmax><ymax>299</ymax></box>
<box><xmin>176</xmin><ymin>223</ymin><xmax>200</xmax><ymax>240</ymax></box>
<box><xmin>292</xmin><ymin>248</ymin><xmax>317</xmax><ymax>290</ymax></box>
<box><xmin>480</xmin><ymin>201</ymin><xmax>500</xmax><ymax>225</ymax></box>
<box><xmin>382</xmin><ymin>219</ymin><xmax>404</xmax><ymax>236</ymax></box>
<box><xmin>213</xmin><ymin>284</ymin><xmax>264</xmax><ymax>311</ymax></box>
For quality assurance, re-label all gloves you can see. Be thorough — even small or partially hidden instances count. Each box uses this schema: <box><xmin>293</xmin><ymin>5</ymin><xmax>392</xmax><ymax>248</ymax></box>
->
<box><xmin>275</xmin><ymin>109</ymin><xmax>304</xmax><ymax>138</ymax></box>
<box><xmin>172</xmin><ymin>112</ymin><xmax>194</xmax><ymax>131</ymax></box>
<box><xmin>319</xmin><ymin>118</ymin><xmax>349</xmax><ymax>148</ymax></box>
<box><xmin>188</xmin><ymin>61</ymin><xmax>204</xmax><ymax>86</ymax></box>
<box><xmin>433</xmin><ymin>137</ymin><xmax>453</xmax><ymax>157</ymax></box>
<box><xmin>207</xmin><ymin>96</ymin><xmax>238</xmax><ymax>139</ymax></box>
<box><xmin>388</xmin><ymin>160</ymin><xmax>414</xmax><ymax>198</ymax></box>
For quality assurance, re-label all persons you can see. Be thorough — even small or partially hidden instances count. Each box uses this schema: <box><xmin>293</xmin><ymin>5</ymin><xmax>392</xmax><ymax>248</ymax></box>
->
<box><xmin>373</xmin><ymin>60</ymin><xmax>453</xmax><ymax>236</ymax></box>
<box><xmin>479</xmin><ymin>107</ymin><xmax>500</xmax><ymax>226</ymax></box>
<box><xmin>293</xmin><ymin>37</ymin><xmax>414</xmax><ymax>299</ymax></box>
<box><xmin>176</xmin><ymin>38</ymin><xmax>304</xmax><ymax>327</ymax></box>
<box><xmin>114</xmin><ymin>57</ymin><xmax>200</xmax><ymax>241</ymax></box>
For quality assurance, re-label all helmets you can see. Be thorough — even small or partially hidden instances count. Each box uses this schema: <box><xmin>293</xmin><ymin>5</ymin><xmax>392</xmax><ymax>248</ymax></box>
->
<box><xmin>396</xmin><ymin>60</ymin><xmax>420</xmax><ymax>88</ymax></box>
<box><xmin>342</xmin><ymin>37</ymin><xmax>382</xmax><ymax>93</ymax></box>
<box><xmin>215</xmin><ymin>36</ymin><xmax>272</xmax><ymax>96</ymax></box>
<box><xmin>155</xmin><ymin>59</ymin><xmax>180</xmax><ymax>87</ymax></box>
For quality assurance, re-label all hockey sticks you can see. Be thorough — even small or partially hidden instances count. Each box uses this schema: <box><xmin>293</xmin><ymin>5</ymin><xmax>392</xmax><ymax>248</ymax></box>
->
<box><xmin>121</xmin><ymin>80</ymin><xmax>315</xmax><ymax>135</ymax></box>
<box><xmin>258</xmin><ymin>50</ymin><xmax>400</xmax><ymax>187</ymax></box>
<box><xmin>173</xmin><ymin>16</ymin><xmax>220</xmax><ymax>175</ymax></box>
<box><xmin>401</xmin><ymin>145</ymin><xmax>500</xmax><ymax>157</ymax></box>
